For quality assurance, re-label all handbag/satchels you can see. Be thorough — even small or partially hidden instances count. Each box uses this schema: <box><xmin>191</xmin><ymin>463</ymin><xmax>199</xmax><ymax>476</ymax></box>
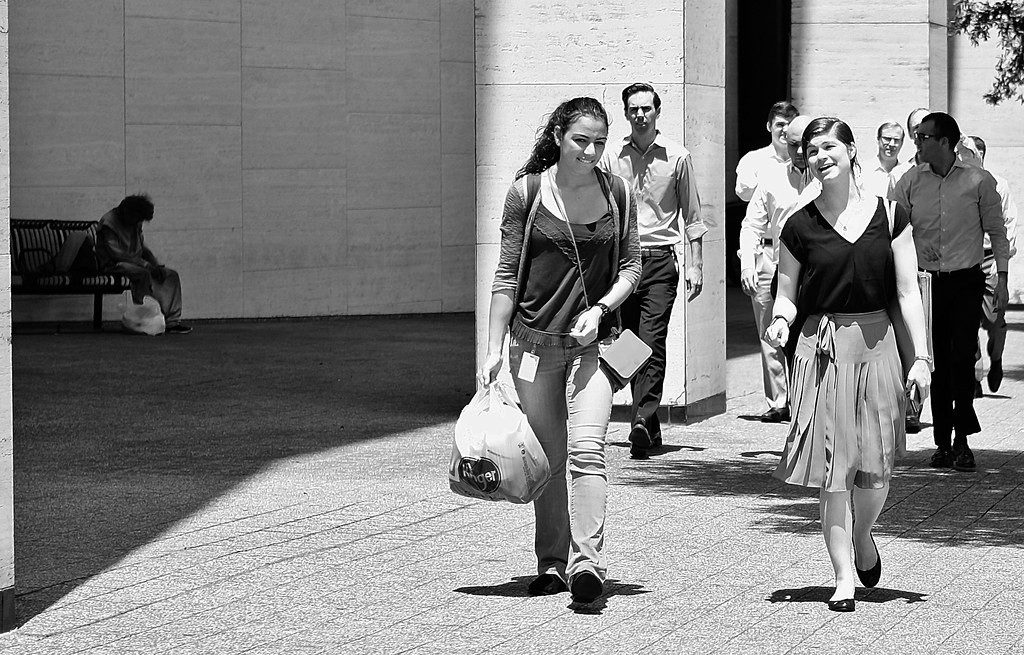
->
<box><xmin>598</xmin><ymin>328</ymin><xmax>653</xmax><ymax>393</ymax></box>
<box><xmin>448</xmin><ymin>379</ymin><xmax>552</xmax><ymax>504</ymax></box>
<box><xmin>120</xmin><ymin>290</ymin><xmax>166</xmax><ymax>336</ymax></box>
<box><xmin>883</xmin><ymin>197</ymin><xmax>935</xmax><ymax>378</ymax></box>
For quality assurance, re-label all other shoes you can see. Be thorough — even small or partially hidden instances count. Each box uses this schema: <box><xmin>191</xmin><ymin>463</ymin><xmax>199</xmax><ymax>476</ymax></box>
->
<box><xmin>529</xmin><ymin>574</ymin><xmax>568</xmax><ymax>596</ymax></box>
<box><xmin>828</xmin><ymin>598</ymin><xmax>856</xmax><ymax>612</ymax></box>
<box><xmin>570</xmin><ymin>570</ymin><xmax>603</xmax><ymax>603</ymax></box>
<box><xmin>973</xmin><ymin>385</ymin><xmax>982</xmax><ymax>398</ymax></box>
<box><xmin>852</xmin><ymin>520</ymin><xmax>881</xmax><ymax>588</ymax></box>
<box><xmin>166</xmin><ymin>325</ymin><xmax>193</xmax><ymax>333</ymax></box>
<box><xmin>988</xmin><ymin>357</ymin><xmax>1003</xmax><ymax>393</ymax></box>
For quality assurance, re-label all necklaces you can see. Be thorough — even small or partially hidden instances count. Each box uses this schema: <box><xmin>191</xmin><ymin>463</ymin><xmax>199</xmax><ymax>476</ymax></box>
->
<box><xmin>818</xmin><ymin>189</ymin><xmax>863</xmax><ymax>231</ymax></box>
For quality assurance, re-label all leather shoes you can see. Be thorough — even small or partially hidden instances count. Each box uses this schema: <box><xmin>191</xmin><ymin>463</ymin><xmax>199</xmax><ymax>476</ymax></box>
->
<box><xmin>628</xmin><ymin>424</ymin><xmax>652</xmax><ymax>458</ymax></box>
<box><xmin>761</xmin><ymin>407</ymin><xmax>789</xmax><ymax>422</ymax></box>
<box><xmin>951</xmin><ymin>443</ymin><xmax>976</xmax><ymax>472</ymax></box>
<box><xmin>651</xmin><ymin>431</ymin><xmax>663</xmax><ymax>448</ymax></box>
<box><xmin>929</xmin><ymin>446</ymin><xmax>951</xmax><ymax>467</ymax></box>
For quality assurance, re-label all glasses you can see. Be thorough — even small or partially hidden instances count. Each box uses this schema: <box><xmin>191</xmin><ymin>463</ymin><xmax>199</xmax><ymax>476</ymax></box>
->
<box><xmin>914</xmin><ymin>132</ymin><xmax>935</xmax><ymax>142</ymax></box>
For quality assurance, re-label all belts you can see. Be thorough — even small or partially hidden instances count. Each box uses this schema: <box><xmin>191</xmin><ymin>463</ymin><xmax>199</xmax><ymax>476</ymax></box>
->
<box><xmin>760</xmin><ymin>238</ymin><xmax>773</xmax><ymax>245</ymax></box>
<box><xmin>984</xmin><ymin>249</ymin><xmax>993</xmax><ymax>256</ymax></box>
<box><xmin>641</xmin><ymin>245</ymin><xmax>671</xmax><ymax>252</ymax></box>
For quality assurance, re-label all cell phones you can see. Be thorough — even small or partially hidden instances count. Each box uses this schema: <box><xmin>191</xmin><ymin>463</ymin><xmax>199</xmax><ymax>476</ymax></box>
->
<box><xmin>910</xmin><ymin>383</ymin><xmax>921</xmax><ymax>413</ymax></box>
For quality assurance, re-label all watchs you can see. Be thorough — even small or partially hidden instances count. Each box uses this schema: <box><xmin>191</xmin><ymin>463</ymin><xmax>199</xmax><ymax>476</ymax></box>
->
<box><xmin>914</xmin><ymin>356</ymin><xmax>935</xmax><ymax>373</ymax></box>
<box><xmin>592</xmin><ymin>303</ymin><xmax>611</xmax><ymax>318</ymax></box>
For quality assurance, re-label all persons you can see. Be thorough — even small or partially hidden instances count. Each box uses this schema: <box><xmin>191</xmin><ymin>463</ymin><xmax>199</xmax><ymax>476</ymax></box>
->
<box><xmin>857</xmin><ymin>121</ymin><xmax>915</xmax><ymax>203</ymax></box>
<box><xmin>478</xmin><ymin>98</ymin><xmax>643</xmax><ymax>607</ymax></box>
<box><xmin>764</xmin><ymin>117</ymin><xmax>934</xmax><ymax>613</ymax></box>
<box><xmin>887</xmin><ymin>107</ymin><xmax>1017</xmax><ymax>473</ymax></box>
<box><xmin>737</xmin><ymin>114</ymin><xmax>823</xmax><ymax>368</ymax></box>
<box><xmin>734</xmin><ymin>101</ymin><xmax>796</xmax><ymax>422</ymax></box>
<box><xmin>95</xmin><ymin>194</ymin><xmax>192</xmax><ymax>334</ymax></box>
<box><xmin>595</xmin><ymin>82</ymin><xmax>708</xmax><ymax>458</ymax></box>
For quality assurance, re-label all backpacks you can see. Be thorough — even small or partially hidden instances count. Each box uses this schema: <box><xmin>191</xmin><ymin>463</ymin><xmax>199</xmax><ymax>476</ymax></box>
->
<box><xmin>56</xmin><ymin>230</ymin><xmax>96</xmax><ymax>277</ymax></box>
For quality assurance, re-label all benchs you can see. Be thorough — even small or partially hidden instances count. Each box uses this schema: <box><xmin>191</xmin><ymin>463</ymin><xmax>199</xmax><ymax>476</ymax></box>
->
<box><xmin>10</xmin><ymin>219</ymin><xmax>132</xmax><ymax>331</ymax></box>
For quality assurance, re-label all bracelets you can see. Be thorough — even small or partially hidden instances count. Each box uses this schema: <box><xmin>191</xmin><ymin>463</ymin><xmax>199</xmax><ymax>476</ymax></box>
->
<box><xmin>769</xmin><ymin>314</ymin><xmax>790</xmax><ymax>327</ymax></box>
